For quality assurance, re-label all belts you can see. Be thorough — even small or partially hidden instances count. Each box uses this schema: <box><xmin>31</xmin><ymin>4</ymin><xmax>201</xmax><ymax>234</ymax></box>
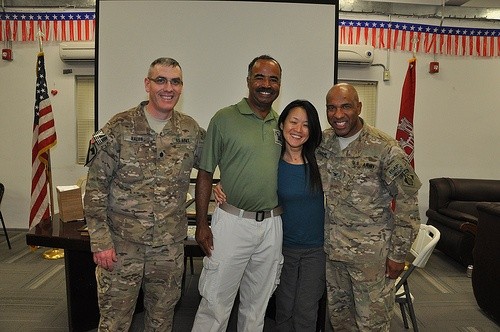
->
<box><xmin>219</xmin><ymin>202</ymin><xmax>283</xmax><ymax>222</ymax></box>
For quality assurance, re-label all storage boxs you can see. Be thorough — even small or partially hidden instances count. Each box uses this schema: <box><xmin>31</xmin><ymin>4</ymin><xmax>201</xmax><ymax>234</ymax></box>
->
<box><xmin>56</xmin><ymin>185</ymin><xmax>83</xmax><ymax>222</ymax></box>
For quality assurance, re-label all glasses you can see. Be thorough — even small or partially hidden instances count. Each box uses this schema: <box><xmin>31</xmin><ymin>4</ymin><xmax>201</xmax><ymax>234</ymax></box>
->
<box><xmin>148</xmin><ymin>76</ymin><xmax>183</xmax><ymax>86</ymax></box>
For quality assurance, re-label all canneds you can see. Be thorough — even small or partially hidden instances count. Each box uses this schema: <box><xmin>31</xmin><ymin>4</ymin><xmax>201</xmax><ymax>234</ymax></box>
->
<box><xmin>467</xmin><ymin>265</ymin><xmax>473</xmax><ymax>277</ymax></box>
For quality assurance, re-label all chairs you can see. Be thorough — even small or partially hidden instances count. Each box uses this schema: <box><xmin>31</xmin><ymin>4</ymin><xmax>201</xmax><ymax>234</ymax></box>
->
<box><xmin>0</xmin><ymin>183</ymin><xmax>12</xmax><ymax>249</ymax></box>
<box><xmin>394</xmin><ymin>224</ymin><xmax>441</xmax><ymax>332</ymax></box>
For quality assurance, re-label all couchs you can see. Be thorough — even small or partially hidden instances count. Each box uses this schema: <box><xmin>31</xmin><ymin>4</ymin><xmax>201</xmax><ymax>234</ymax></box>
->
<box><xmin>426</xmin><ymin>177</ymin><xmax>500</xmax><ymax>273</ymax></box>
<box><xmin>472</xmin><ymin>202</ymin><xmax>500</xmax><ymax>326</ymax></box>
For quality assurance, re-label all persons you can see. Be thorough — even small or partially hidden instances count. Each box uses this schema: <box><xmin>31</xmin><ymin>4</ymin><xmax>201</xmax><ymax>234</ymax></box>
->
<box><xmin>314</xmin><ymin>83</ymin><xmax>423</xmax><ymax>332</ymax></box>
<box><xmin>213</xmin><ymin>100</ymin><xmax>326</xmax><ymax>332</ymax></box>
<box><xmin>84</xmin><ymin>58</ymin><xmax>207</xmax><ymax>332</ymax></box>
<box><xmin>192</xmin><ymin>55</ymin><xmax>284</xmax><ymax>332</ymax></box>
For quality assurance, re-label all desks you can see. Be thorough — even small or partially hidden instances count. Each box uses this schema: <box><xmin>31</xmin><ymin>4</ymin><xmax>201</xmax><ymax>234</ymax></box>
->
<box><xmin>25</xmin><ymin>183</ymin><xmax>217</xmax><ymax>332</ymax></box>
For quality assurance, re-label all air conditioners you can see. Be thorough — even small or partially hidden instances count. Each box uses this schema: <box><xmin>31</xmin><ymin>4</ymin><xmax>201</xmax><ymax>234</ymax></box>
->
<box><xmin>60</xmin><ymin>42</ymin><xmax>95</xmax><ymax>61</ymax></box>
<box><xmin>338</xmin><ymin>44</ymin><xmax>375</xmax><ymax>67</ymax></box>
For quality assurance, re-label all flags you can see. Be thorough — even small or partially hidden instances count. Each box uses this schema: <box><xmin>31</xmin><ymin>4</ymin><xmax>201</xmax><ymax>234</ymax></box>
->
<box><xmin>391</xmin><ymin>59</ymin><xmax>416</xmax><ymax>213</ymax></box>
<box><xmin>29</xmin><ymin>52</ymin><xmax>57</xmax><ymax>230</ymax></box>
